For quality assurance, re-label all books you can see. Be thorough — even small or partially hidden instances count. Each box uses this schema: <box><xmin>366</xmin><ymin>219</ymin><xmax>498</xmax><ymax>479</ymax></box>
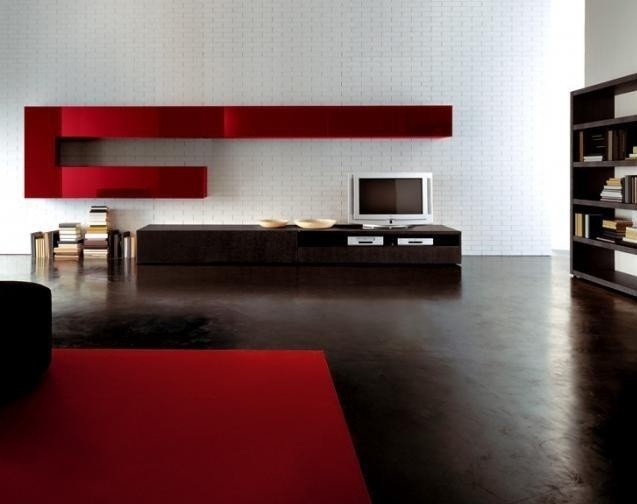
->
<box><xmin>28</xmin><ymin>260</ymin><xmax>138</xmax><ymax>289</ymax></box>
<box><xmin>29</xmin><ymin>202</ymin><xmax>137</xmax><ymax>260</ymax></box>
<box><xmin>572</xmin><ymin>125</ymin><xmax>636</xmax><ymax>252</ymax></box>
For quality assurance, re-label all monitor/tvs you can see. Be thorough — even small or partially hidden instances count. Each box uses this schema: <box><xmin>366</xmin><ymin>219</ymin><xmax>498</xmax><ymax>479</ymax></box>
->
<box><xmin>353</xmin><ymin>173</ymin><xmax>428</xmax><ymax>229</ymax></box>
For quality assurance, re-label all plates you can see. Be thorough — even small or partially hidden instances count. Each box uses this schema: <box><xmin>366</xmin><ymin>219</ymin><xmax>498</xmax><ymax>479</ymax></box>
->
<box><xmin>292</xmin><ymin>218</ymin><xmax>336</xmax><ymax>229</ymax></box>
<box><xmin>258</xmin><ymin>218</ymin><xmax>287</xmax><ymax>228</ymax></box>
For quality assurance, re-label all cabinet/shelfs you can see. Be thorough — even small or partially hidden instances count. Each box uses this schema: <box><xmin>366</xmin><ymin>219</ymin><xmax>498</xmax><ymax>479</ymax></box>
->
<box><xmin>568</xmin><ymin>73</ymin><xmax>635</xmax><ymax>300</ymax></box>
